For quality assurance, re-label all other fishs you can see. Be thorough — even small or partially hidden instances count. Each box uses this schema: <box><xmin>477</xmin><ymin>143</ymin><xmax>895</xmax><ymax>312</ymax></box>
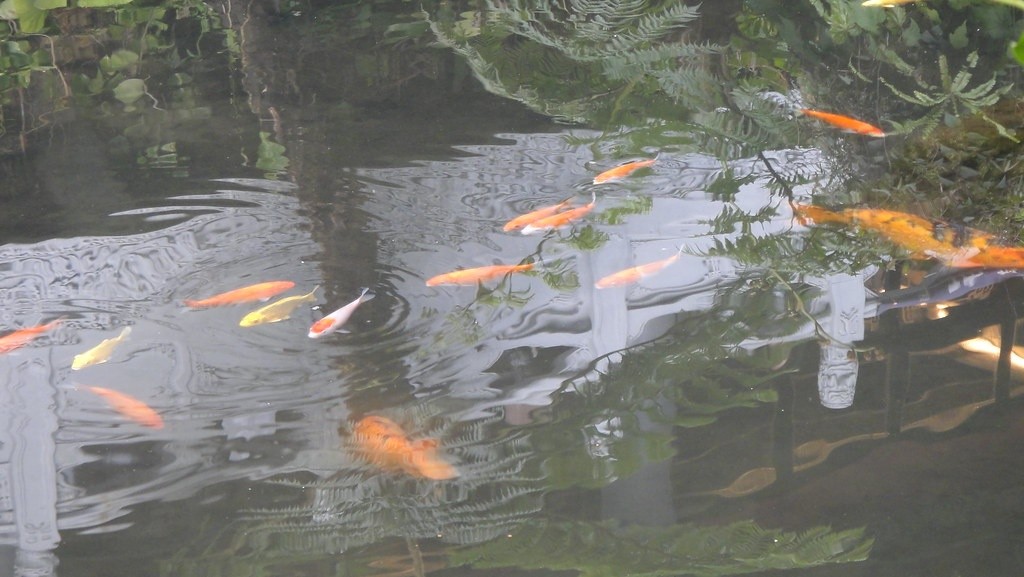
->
<box><xmin>350</xmin><ymin>415</ymin><xmax>461</xmax><ymax>481</ymax></box>
<box><xmin>594</xmin><ymin>255</ymin><xmax>677</xmax><ymax>290</ymax></box>
<box><xmin>425</xmin><ymin>264</ymin><xmax>534</xmax><ymax>287</ymax></box>
<box><xmin>795</xmin><ymin>202</ymin><xmax>1024</xmax><ymax>269</ymax></box>
<box><xmin>502</xmin><ymin>160</ymin><xmax>659</xmax><ymax>237</ymax></box>
<box><xmin>0</xmin><ymin>318</ymin><xmax>164</xmax><ymax>432</ymax></box>
<box><xmin>182</xmin><ymin>280</ymin><xmax>376</xmax><ymax>340</ymax></box>
<box><xmin>800</xmin><ymin>108</ymin><xmax>885</xmax><ymax>138</ymax></box>
